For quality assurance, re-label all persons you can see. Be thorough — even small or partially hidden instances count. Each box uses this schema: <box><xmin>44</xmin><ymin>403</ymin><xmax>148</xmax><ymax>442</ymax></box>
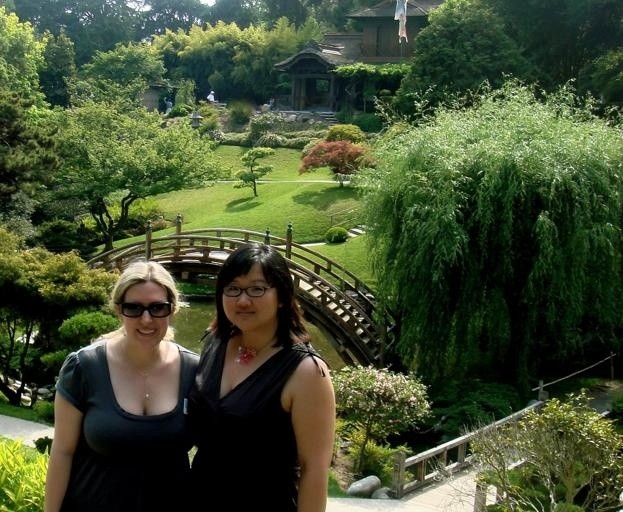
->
<box><xmin>206</xmin><ymin>90</ymin><xmax>214</xmax><ymax>103</ymax></box>
<box><xmin>44</xmin><ymin>254</ymin><xmax>206</xmax><ymax>511</ymax></box>
<box><xmin>163</xmin><ymin>97</ymin><xmax>172</xmax><ymax>114</ymax></box>
<box><xmin>185</xmin><ymin>239</ymin><xmax>335</xmax><ymax>511</ymax></box>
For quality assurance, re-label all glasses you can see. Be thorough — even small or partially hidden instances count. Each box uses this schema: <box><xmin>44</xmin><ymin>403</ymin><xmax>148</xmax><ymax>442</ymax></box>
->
<box><xmin>222</xmin><ymin>285</ymin><xmax>274</xmax><ymax>298</ymax></box>
<box><xmin>117</xmin><ymin>297</ymin><xmax>173</xmax><ymax>318</ymax></box>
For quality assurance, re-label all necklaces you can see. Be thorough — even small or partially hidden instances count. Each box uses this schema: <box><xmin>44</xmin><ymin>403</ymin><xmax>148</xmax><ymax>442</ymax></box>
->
<box><xmin>231</xmin><ymin>325</ymin><xmax>279</xmax><ymax>366</ymax></box>
